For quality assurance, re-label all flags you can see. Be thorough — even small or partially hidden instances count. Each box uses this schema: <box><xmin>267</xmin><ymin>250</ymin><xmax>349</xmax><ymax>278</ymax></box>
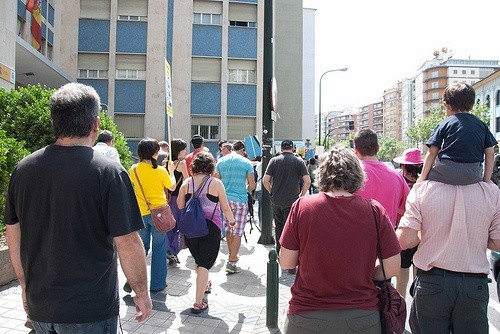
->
<box><xmin>26</xmin><ymin>0</ymin><xmax>42</xmax><ymax>52</ymax></box>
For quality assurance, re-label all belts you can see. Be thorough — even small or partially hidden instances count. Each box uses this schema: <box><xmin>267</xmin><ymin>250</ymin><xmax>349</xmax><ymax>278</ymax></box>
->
<box><xmin>416</xmin><ymin>268</ymin><xmax>487</xmax><ymax>278</ymax></box>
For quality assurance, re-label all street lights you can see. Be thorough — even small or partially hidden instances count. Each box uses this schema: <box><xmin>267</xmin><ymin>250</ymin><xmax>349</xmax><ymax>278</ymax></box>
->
<box><xmin>323</xmin><ymin>127</ymin><xmax>356</xmax><ymax>149</ymax></box>
<box><xmin>317</xmin><ymin>67</ymin><xmax>347</xmax><ymax>153</ymax></box>
<box><xmin>324</xmin><ymin>107</ymin><xmax>344</xmax><ymax>151</ymax></box>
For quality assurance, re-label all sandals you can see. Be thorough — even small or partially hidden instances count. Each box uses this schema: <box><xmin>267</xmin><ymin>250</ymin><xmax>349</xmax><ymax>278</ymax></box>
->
<box><xmin>205</xmin><ymin>281</ymin><xmax>211</xmax><ymax>293</ymax></box>
<box><xmin>192</xmin><ymin>302</ymin><xmax>208</xmax><ymax>312</ymax></box>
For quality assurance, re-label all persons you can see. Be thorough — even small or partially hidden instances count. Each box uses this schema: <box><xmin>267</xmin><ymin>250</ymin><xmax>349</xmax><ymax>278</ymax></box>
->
<box><xmin>185</xmin><ymin>135</ymin><xmax>204</xmax><ymax>176</ymax></box>
<box><xmin>162</xmin><ymin>138</ymin><xmax>189</xmax><ymax>264</ymax></box>
<box><xmin>91</xmin><ymin>130</ymin><xmax>120</xmax><ymax>163</ymax></box>
<box><xmin>4</xmin><ymin>82</ymin><xmax>153</xmax><ymax>334</ymax></box>
<box><xmin>416</xmin><ymin>82</ymin><xmax>498</xmax><ymax>185</ymax></box>
<box><xmin>156</xmin><ymin>141</ymin><xmax>170</xmax><ymax>166</ymax></box>
<box><xmin>216</xmin><ymin>140</ymin><xmax>233</xmax><ymax>161</ymax></box>
<box><xmin>481</xmin><ymin>145</ymin><xmax>500</xmax><ymax>302</ymax></box>
<box><xmin>262</xmin><ymin>139</ymin><xmax>311</xmax><ymax>256</ymax></box>
<box><xmin>396</xmin><ymin>179</ymin><xmax>500</xmax><ymax>334</ymax></box>
<box><xmin>214</xmin><ymin>141</ymin><xmax>256</xmax><ymax>273</ymax></box>
<box><xmin>243</xmin><ymin>150</ymin><xmax>276</xmax><ymax>231</ymax></box>
<box><xmin>279</xmin><ymin>142</ymin><xmax>401</xmax><ymax>334</ymax></box>
<box><xmin>123</xmin><ymin>137</ymin><xmax>176</xmax><ymax>295</ymax></box>
<box><xmin>397</xmin><ymin>148</ymin><xmax>424</xmax><ymax>299</ymax></box>
<box><xmin>353</xmin><ymin>128</ymin><xmax>411</xmax><ymax>231</ymax></box>
<box><xmin>293</xmin><ymin>153</ymin><xmax>320</xmax><ymax>196</ymax></box>
<box><xmin>177</xmin><ymin>152</ymin><xmax>237</xmax><ymax>314</ymax></box>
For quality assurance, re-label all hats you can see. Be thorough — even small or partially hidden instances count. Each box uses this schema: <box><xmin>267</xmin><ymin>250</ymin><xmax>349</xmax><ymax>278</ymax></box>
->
<box><xmin>281</xmin><ymin>140</ymin><xmax>293</xmax><ymax>147</ymax></box>
<box><xmin>393</xmin><ymin>149</ymin><xmax>424</xmax><ymax>164</ymax></box>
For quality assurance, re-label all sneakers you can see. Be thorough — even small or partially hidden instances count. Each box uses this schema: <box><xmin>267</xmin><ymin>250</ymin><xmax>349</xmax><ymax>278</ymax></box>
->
<box><xmin>228</xmin><ymin>257</ymin><xmax>239</xmax><ymax>261</ymax></box>
<box><xmin>225</xmin><ymin>262</ymin><xmax>241</xmax><ymax>273</ymax></box>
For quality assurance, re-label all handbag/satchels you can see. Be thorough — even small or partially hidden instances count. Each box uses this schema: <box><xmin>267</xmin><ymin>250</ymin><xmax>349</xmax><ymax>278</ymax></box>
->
<box><xmin>150</xmin><ymin>204</ymin><xmax>176</xmax><ymax>232</ymax></box>
<box><xmin>376</xmin><ymin>281</ymin><xmax>406</xmax><ymax>334</ymax></box>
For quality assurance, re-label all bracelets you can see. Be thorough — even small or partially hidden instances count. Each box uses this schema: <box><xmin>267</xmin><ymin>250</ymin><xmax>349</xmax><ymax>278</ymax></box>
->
<box><xmin>228</xmin><ymin>219</ymin><xmax>236</xmax><ymax>227</ymax></box>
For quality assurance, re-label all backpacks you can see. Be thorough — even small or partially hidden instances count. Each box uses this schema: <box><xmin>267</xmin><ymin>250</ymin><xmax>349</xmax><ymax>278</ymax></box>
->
<box><xmin>164</xmin><ymin>160</ymin><xmax>183</xmax><ymax>202</ymax></box>
<box><xmin>176</xmin><ymin>176</ymin><xmax>218</xmax><ymax>239</ymax></box>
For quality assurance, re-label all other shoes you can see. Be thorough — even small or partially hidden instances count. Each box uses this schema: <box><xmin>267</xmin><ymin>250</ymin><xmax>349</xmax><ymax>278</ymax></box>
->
<box><xmin>123</xmin><ymin>282</ymin><xmax>132</xmax><ymax>293</ymax></box>
<box><xmin>24</xmin><ymin>315</ymin><xmax>34</xmax><ymax>330</ymax></box>
<box><xmin>288</xmin><ymin>269</ymin><xmax>296</xmax><ymax>274</ymax></box>
<box><xmin>167</xmin><ymin>250</ymin><xmax>180</xmax><ymax>264</ymax></box>
